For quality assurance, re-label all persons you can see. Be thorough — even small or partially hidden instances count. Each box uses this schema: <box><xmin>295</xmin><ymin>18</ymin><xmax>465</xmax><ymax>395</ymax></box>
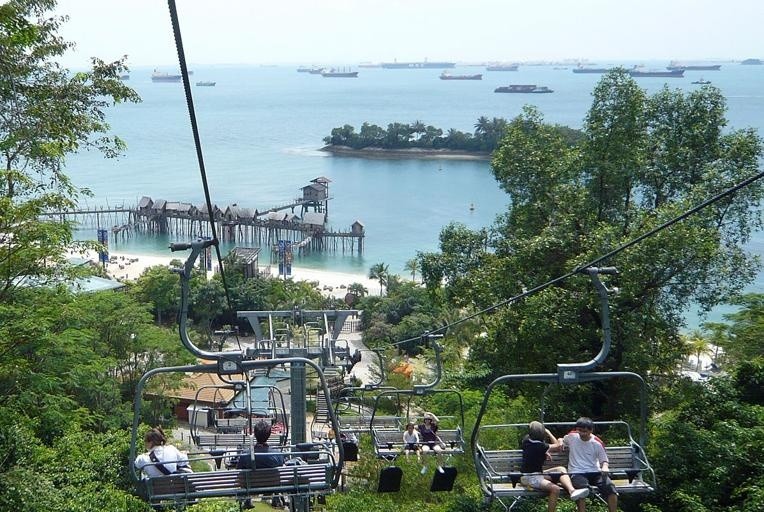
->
<box><xmin>412</xmin><ymin>412</ymin><xmax>446</xmax><ymax>474</ymax></box>
<box><xmin>403</xmin><ymin>423</ymin><xmax>421</xmax><ymax>463</ymax></box>
<box><xmin>558</xmin><ymin>417</ymin><xmax>619</xmax><ymax>512</ymax></box>
<box><xmin>520</xmin><ymin>419</ymin><xmax>589</xmax><ymax>512</ymax></box>
<box><xmin>134</xmin><ymin>425</ymin><xmax>196</xmax><ymax>512</ymax></box>
<box><xmin>237</xmin><ymin>420</ymin><xmax>285</xmax><ymax>509</ymax></box>
<box><xmin>326</xmin><ymin>428</ymin><xmax>336</xmax><ymax>455</ymax></box>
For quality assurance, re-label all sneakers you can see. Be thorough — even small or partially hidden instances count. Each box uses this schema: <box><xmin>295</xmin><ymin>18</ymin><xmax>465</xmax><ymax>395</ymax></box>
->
<box><xmin>242</xmin><ymin>500</ymin><xmax>251</xmax><ymax>509</ymax></box>
<box><xmin>570</xmin><ymin>488</ymin><xmax>590</xmax><ymax>501</ymax></box>
<box><xmin>421</xmin><ymin>466</ymin><xmax>427</xmax><ymax>474</ymax></box>
<box><xmin>436</xmin><ymin>465</ymin><xmax>444</xmax><ymax>473</ymax></box>
<box><xmin>270</xmin><ymin>500</ymin><xmax>281</xmax><ymax>507</ymax></box>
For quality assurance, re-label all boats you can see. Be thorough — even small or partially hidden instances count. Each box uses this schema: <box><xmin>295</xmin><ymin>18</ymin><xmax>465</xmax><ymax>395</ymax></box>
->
<box><xmin>573</xmin><ymin>66</ymin><xmax>612</xmax><ymax>75</ymax></box>
<box><xmin>629</xmin><ymin>70</ymin><xmax>685</xmax><ymax>78</ymax></box>
<box><xmin>741</xmin><ymin>58</ymin><xmax>763</xmax><ymax>65</ymax></box>
<box><xmin>196</xmin><ymin>81</ymin><xmax>216</xmax><ymax>87</ymax></box>
<box><xmin>296</xmin><ymin>64</ymin><xmax>358</xmax><ymax>78</ymax></box>
<box><xmin>151</xmin><ymin>72</ymin><xmax>182</xmax><ymax>81</ymax></box>
<box><xmin>380</xmin><ymin>61</ymin><xmax>456</xmax><ymax>69</ymax></box>
<box><xmin>486</xmin><ymin>66</ymin><xmax>519</xmax><ymax>71</ymax></box>
<box><xmin>666</xmin><ymin>64</ymin><xmax>721</xmax><ymax>71</ymax></box>
<box><xmin>438</xmin><ymin>72</ymin><xmax>482</xmax><ymax>80</ymax></box>
<box><xmin>494</xmin><ymin>84</ymin><xmax>554</xmax><ymax>94</ymax></box>
